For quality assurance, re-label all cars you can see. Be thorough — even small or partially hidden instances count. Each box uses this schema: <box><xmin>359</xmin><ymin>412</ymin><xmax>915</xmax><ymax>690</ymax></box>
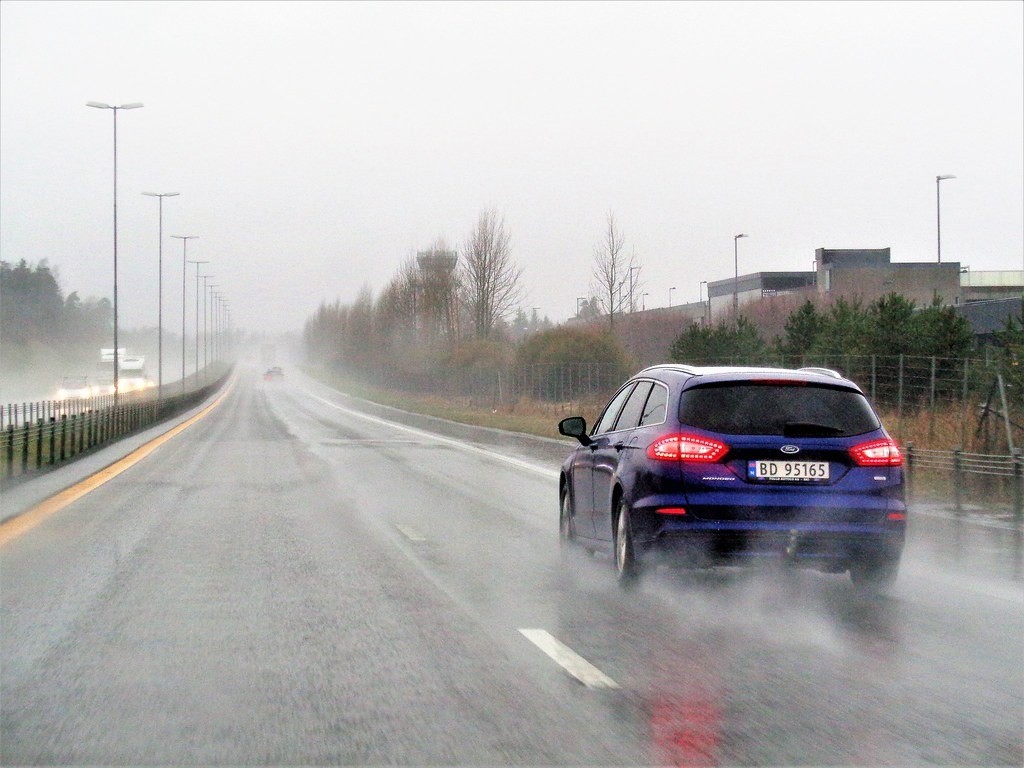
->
<box><xmin>557</xmin><ymin>364</ymin><xmax>908</xmax><ymax>598</ymax></box>
<box><xmin>53</xmin><ymin>348</ymin><xmax>154</xmax><ymax>408</ymax></box>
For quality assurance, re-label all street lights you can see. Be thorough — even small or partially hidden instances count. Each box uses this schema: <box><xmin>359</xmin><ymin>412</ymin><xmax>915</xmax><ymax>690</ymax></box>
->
<box><xmin>935</xmin><ymin>175</ymin><xmax>958</xmax><ymax>303</ymax></box>
<box><xmin>732</xmin><ymin>234</ymin><xmax>748</xmax><ymax>325</ymax></box>
<box><xmin>207</xmin><ymin>284</ymin><xmax>234</xmax><ymax>375</ymax></box>
<box><xmin>669</xmin><ymin>287</ymin><xmax>676</xmax><ymax>307</ymax></box>
<box><xmin>629</xmin><ymin>267</ymin><xmax>638</xmax><ymax>313</ymax></box>
<box><xmin>171</xmin><ymin>234</ymin><xmax>201</xmax><ymax>397</ymax></box>
<box><xmin>185</xmin><ymin>259</ymin><xmax>211</xmax><ymax>388</ymax></box>
<box><xmin>85</xmin><ymin>101</ymin><xmax>145</xmax><ymax>414</ymax></box>
<box><xmin>642</xmin><ymin>293</ymin><xmax>649</xmax><ymax>310</ymax></box>
<box><xmin>700</xmin><ymin>281</ymin><xmax>707</xmax><ymax>301</ymax></box>
<box><xmin>195</xmin><ymin>274</ymin><xmax>216</xmax><ymax>386</ymax></box>
<box><xmin>139</xmin><ymin>190</ymin><xmax>180</xmax><ymax>402</ymax></box>
<box><xmin>577</xmin><ymin>297</ymin><xmax>586</xmax><ymax>321</ymax></box>
